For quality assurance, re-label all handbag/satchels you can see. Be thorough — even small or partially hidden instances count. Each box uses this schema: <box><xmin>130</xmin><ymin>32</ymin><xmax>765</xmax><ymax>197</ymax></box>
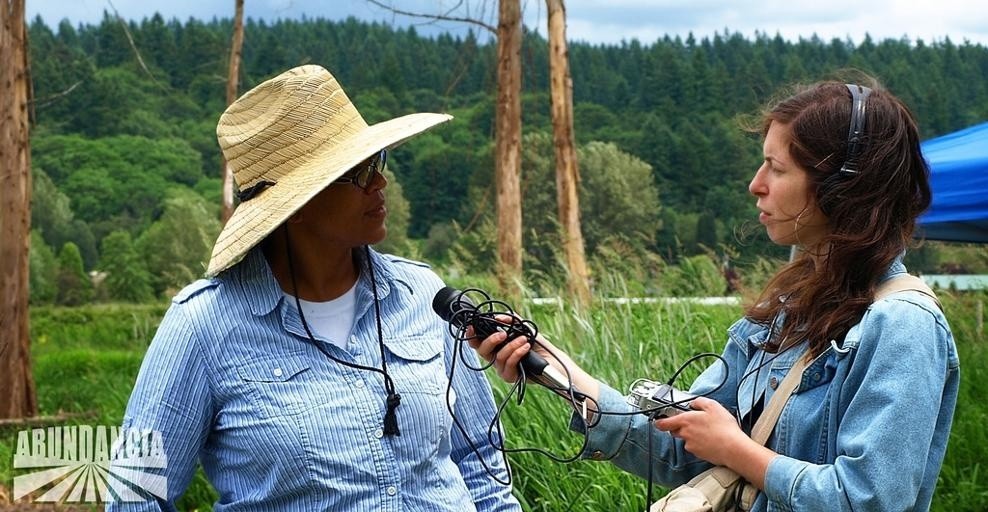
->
<box><xmin>645</xmin><ymin>464</ymin><xmax>758</xmax><ymax>512</ymax></box>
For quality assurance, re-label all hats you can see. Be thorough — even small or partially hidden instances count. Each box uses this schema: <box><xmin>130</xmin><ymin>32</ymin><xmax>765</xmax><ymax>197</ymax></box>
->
<box><xmin>206</xmin><ymin>64</ymin><xmax>454</xmax><ymax>278</ymax></box>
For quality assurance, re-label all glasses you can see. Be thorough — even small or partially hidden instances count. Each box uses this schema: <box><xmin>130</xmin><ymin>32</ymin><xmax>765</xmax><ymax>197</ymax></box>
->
<box><xmin>336</xmin><ymin>150</ymin><xmax>387</xmax><ymax>190</ymax></box>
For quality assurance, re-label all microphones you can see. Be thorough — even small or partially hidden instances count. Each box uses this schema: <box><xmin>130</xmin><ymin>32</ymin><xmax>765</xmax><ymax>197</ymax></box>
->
<box><xmin>433</xmin><ymin>287</ymin><xmax>586</xmax><ymax>401</ymax></box>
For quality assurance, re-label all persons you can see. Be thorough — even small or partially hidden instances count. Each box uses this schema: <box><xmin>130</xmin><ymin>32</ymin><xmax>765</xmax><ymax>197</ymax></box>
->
<box><xmin>100</xmin><ymin>61</ymin><xmax>521</xmax><ymax>511</ymax></box>
<box><xmin>465</xmin><ymin>64</ymin><xmax>962</xmax><ymax>510</ymax></box>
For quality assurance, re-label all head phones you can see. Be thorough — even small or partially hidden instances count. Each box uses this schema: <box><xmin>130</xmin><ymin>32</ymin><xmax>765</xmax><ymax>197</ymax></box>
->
<box><xmin>816</xmin><ymin>84</ymin><xmax>873</xmax><ymax>219</ymax></box>
